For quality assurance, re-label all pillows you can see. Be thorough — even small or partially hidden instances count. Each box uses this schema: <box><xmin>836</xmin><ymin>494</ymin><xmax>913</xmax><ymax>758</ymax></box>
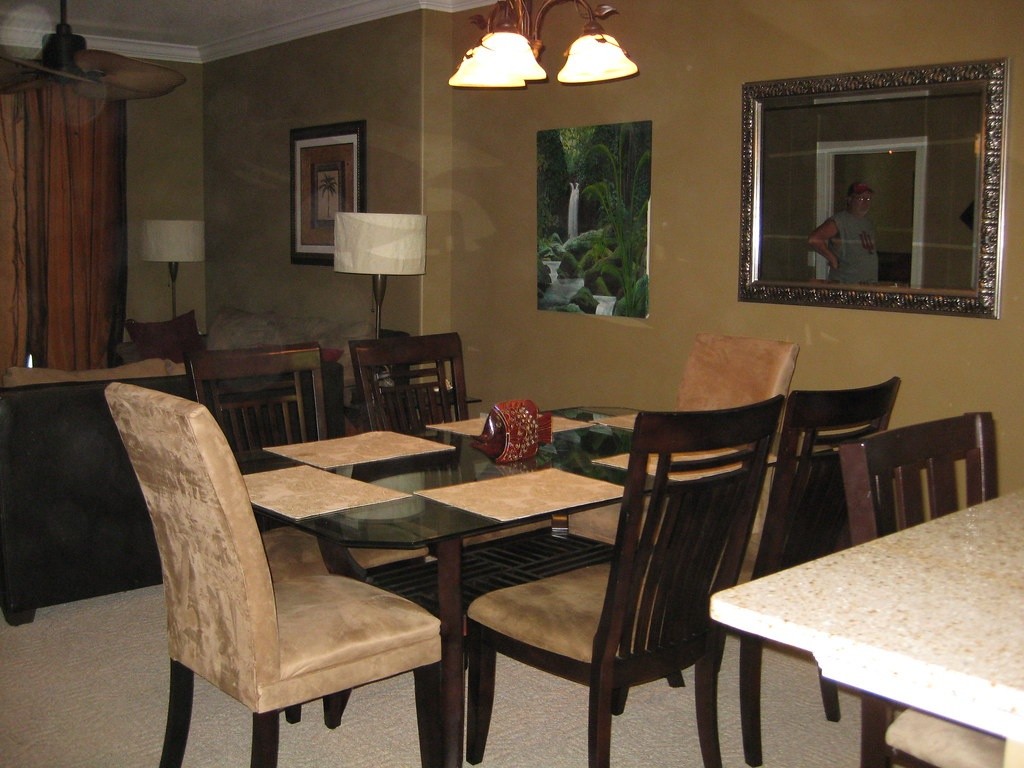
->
<box><xmin>125</xmin><ymin>309</ymin><xmax>206</xmax><ymax>365</ymax></box>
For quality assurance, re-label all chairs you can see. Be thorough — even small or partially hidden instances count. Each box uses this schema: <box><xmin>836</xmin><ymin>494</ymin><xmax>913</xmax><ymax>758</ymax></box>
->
<box><xmin>104</xmin><ymin>331</ymin><xmax>1001</xmax><ymax>768</ymax></box>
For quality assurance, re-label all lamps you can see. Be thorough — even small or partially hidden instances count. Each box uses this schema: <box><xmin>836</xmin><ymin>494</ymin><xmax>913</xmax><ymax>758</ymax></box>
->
<box><xmin>449</xmin><ymin>1</ymin><xmax>639</xmax><ymax>90</ymax></box>
<box><xmin>333</xmin><ymin>213</ymin><xmax>428</xmax><ymax>337</ymax></box>
<box><xmin>142</xmin><ymin>219</ymin><xmax>205</xmax><ymax>318</ymax></box>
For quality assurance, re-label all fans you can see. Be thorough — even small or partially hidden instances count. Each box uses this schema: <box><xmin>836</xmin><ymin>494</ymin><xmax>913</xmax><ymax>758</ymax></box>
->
<box><xmin>0</xmin><ymin>0</ymin><xmax>186</xmax><ymax>105</ymax></box>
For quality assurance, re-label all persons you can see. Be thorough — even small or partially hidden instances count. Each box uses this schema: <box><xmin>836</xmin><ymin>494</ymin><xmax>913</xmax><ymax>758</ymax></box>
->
<box><xmin>808</xmin><ymin>182</ymin><xmax>878</xmax><ymax>283</ymax></box>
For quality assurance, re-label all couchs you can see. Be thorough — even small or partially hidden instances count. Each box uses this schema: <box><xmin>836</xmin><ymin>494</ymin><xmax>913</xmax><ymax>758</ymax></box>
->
<box><xmin>1</xmin><ymin>309</ymin><xmax>413</xmax><ymax>627</ymax></box>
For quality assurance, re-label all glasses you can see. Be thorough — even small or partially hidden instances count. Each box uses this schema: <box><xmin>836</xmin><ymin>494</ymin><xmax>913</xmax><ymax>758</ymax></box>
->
<box><xmin>854</xmin><ymin>196</ymin><xmax>872</xmax><ymax>201</ymax></box>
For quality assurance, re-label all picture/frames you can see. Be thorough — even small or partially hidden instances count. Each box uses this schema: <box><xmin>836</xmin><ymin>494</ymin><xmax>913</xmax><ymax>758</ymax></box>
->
<box><xmin>290</xmin><ymin>119</ymin><xmax>367</xmax><ymax>265</ymax></box>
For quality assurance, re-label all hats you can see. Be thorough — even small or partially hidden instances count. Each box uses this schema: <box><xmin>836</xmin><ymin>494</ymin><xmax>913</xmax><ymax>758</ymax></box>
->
<box><xmin>848</xmin><ymin>183</ymin><xmax>875</xmax><ymax>194</ymax></box>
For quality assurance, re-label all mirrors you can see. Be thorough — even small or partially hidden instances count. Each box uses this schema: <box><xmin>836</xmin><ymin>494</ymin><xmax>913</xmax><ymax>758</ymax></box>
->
<box><xmin>736</xmin><ymin>55</ymin><xmax>1011</xmax><ymax>320</ymax></box>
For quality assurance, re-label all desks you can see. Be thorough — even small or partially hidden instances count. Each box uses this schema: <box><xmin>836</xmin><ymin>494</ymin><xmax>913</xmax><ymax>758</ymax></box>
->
<box><xmin>711</xmin><ymin>487</ymin><xmax>1024</xmax><ymax>768</ymax></box>
<box><xmin>233</xmin><ymin>403</ymin><xmax>834</xmax><ymax>767</ymax></box>
